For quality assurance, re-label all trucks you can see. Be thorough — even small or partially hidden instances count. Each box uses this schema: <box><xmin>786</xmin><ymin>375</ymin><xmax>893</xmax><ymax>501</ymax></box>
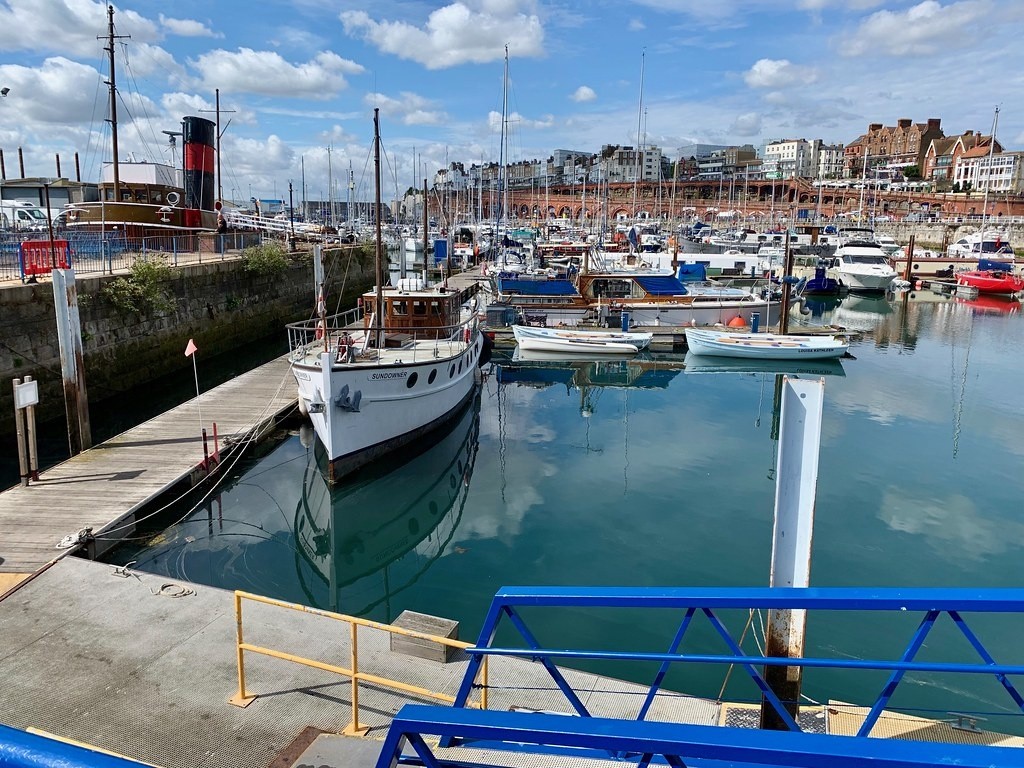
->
<box><xmin>0</xmin><ymin>200</ymin><xmax>49</xmax><ymax>232</ymax></box>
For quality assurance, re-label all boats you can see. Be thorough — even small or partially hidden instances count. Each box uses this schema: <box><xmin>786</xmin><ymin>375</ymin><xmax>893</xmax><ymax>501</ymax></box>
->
<box><xmin>684</xmin><ymin>327</ymin><xmax>849</xmax><ymax>360</ymax></box>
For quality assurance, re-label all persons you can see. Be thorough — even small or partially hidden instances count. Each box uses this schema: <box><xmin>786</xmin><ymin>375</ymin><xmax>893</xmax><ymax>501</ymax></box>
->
<box><xmin>215</xmin><ymin>214</ymin><xmax>227</xmax><ymax>254</ymax></box>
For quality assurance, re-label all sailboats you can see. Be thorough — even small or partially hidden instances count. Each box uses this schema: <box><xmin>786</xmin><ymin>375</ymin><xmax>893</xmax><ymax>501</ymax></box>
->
<box><xmin>64</xmin><ymin>0</ymin><xmax>227</xmax><ymax>253</ymax></box>
<box><xmin>301</xmin><ymin>42</ymin><xmax>1024</xmax><ymax>329</ymax></box>
<box><xmin>283</xmin><ymin>107</ymin><xmax>484</xmax><ymax>471</ymax></box>
<box><xmin>292</xmin><ymin>386</ymin><xmax>482</xmax><ymax>627</ymax></box>
